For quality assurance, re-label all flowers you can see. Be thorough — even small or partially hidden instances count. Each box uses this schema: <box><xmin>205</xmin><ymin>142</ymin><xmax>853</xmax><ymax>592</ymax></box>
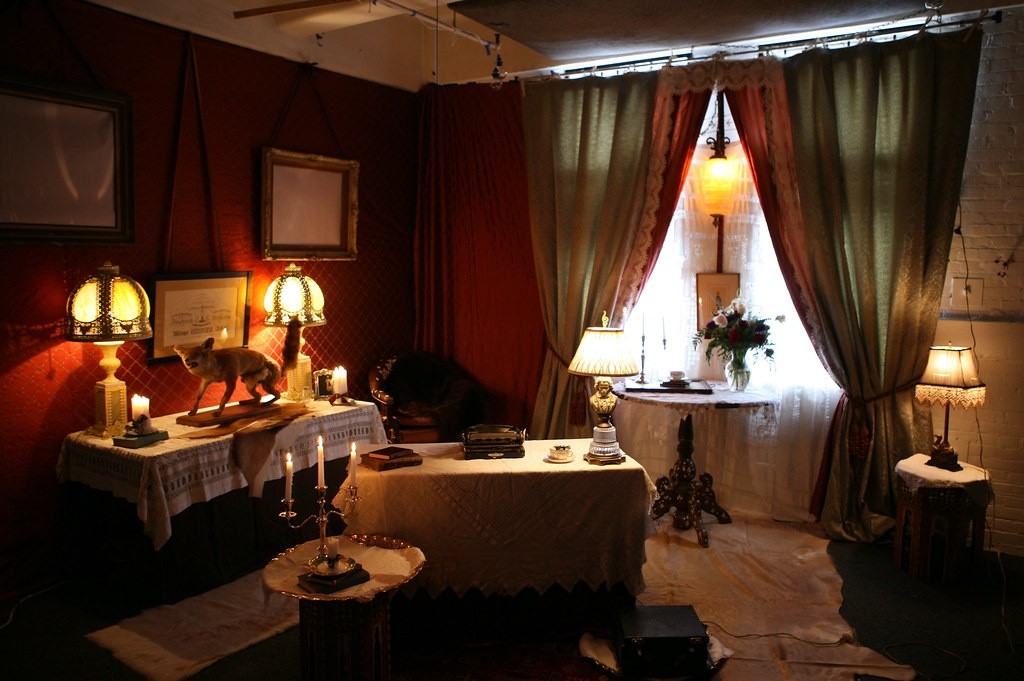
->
<box><xmin>692</xmin><ymin>297</ymin><xmax>786</xmax><ymax>390</ymax></box>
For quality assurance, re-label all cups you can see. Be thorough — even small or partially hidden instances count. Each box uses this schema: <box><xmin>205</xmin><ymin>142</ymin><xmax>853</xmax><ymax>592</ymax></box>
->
<box><xmin>550</xmin><ymin>445</ymin><xmax>573</xmax><ymax>459</ymax></box>
<box><xmin>671</xmin><ymin>371</ymin><xmax>686</xmax><ymax>380</ymax></box>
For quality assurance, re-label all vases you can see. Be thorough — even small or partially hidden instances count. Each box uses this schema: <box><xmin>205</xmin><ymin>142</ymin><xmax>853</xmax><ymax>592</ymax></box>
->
<box><xmin>724</xmin><ymin>348</ymin><xmax>750</xmax><ymax>392</ymax></box>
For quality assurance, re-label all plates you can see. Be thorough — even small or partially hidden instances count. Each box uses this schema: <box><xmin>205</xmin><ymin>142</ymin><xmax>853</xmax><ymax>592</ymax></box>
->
<box><xmin>545</xmin><ymin>455</ymin><xmax>576</xmax><ymax>462</ymax></box>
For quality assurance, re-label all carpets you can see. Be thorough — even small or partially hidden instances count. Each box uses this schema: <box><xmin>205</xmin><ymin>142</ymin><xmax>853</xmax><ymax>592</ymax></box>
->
<box><xmin>83</xmin><ymin>567</ymin><xmax>299</xmax><ymax>681</ymax></box>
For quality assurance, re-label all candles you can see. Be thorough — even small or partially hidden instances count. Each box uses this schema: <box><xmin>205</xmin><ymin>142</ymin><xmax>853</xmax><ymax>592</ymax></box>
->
<box><xmin>131</xmin><ymin>393</ymin><xmax>152</xmax><ymax>432</ymax></box>
<box><xmin>332</xmin><ymin>365</ymin><xmax>347</xmax><ymax>394</ymax></box>
<box><xmin>642</xmin><ymin>311</ymin><xmax>644</xmax><ymax>336</ymax></box>
<box><xmin>350</xmin><ymin>441</ymin><xmax>356</xmax><ymax>486</ymax></box>
<box><xmin>285</xmin><ymin>453</ymin><xmax>293</xmax><ymax>500</ymax></box>
<box><xmin>317</xmin><ymin>436</ymin><xmax>323</xmax><ymax>487</ymax></box>
<box><xmin>663</xmin><ymin>316</ymin><xmax>665</xmax><ymax>339</ymax></box>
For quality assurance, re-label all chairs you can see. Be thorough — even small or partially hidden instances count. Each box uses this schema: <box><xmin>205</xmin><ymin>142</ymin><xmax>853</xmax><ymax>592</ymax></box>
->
<box><xmin>367</xmin><ymin>351</ymin><xmax>505</xmax><ymax>444</ymax></box>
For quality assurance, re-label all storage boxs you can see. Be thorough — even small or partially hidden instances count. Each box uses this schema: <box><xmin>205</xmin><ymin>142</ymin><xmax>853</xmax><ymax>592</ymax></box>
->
<box><xmin>608</xmin><ymin>606</ymin><xmax>709</xmax><ymax>681</ymax></box>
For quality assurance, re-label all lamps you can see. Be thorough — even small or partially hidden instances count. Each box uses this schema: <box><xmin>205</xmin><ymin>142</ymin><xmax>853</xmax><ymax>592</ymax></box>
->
<box><xmin>567</xmin><ymin>311</ymin><xmax>640</xmax><ymax>466</ymax></box>
<box><xmin>702</xmin><ymin>130</ymin><xmax>734</xmax><ymax>229</ymax></box>
<box><xmin>263</xmin><ymin>262</ymin><xmax>328</xmax><ymax>402</ymax></box>
<box><xmin>915</xmin><ymin>346</ymin><xmax>986</xmax><ymax>472</ymax></box>
<box><xmin>66</xmin><ymin>260</ymin><xmax>155</xmax><ymax>441</ymax></box>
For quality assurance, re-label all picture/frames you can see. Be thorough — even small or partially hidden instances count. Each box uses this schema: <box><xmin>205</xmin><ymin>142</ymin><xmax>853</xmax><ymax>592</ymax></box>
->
<box><xmin>252</xmin><ymin>146</ymin><xmax>359</xmax><ymax>261</ymax></box>
<box><xmin>696</xmin><ymin>272</ymin><xmax>740</xmax><ymax>331</ymax></box>
<box><xmin>0</xmin><ymin>74</ymin><xmax>137</xmax><ymax>248</ymax></box>
<box><xmin>313</xmin><ymin>368</ymin><xmax>334</xmax><ymax>401</ymax></box>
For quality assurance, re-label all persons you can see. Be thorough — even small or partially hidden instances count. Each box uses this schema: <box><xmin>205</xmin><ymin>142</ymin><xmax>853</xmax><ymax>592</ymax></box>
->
<box><xmin>590</xmin><ymin>377</ymin><xmax>618</xmax><ymax>415</ymax></box>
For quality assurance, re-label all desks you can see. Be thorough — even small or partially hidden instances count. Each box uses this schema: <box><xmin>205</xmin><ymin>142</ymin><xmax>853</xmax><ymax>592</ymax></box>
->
<box><xmin>893</xmin><ymin>453</ymin><xmax>996</xmax><ymax>579</ymax></box>
<box><xmin>54</xmin><ymin>390</ymin><xmax>388</xmax><ymax>603</ymax></box>
<box><xmin>612</xmin><ymin>378</ymin><xmax>781</xmax><ymax>549</ymax></box>
<box><xmin>331</xmin><ymin>438</ymin><xmax>660</xmax><ymax>624</ymax></box>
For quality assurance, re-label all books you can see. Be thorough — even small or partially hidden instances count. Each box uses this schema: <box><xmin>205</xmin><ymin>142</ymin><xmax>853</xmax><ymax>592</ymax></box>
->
<box><xmin>358</xmin><ymin>446</ymin><xmax>423</xmax><ymax>471</ymax></box>
<box><xmin>112</xmin><ymin>430</ymin><xmax>169</xmax><ymax>448</ymax></box>
